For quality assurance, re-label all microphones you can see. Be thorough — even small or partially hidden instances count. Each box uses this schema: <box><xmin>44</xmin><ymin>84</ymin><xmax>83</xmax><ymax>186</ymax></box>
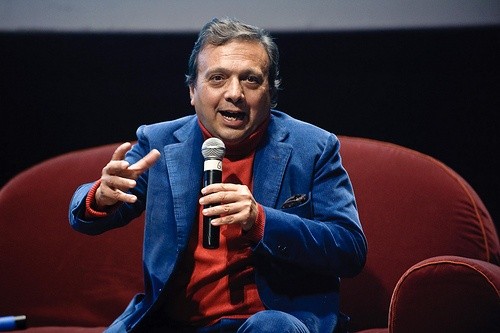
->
<box><xmin>201</xmin><ymin>137</ymin><xmax>226</xmax><ymax>249</ymax></box>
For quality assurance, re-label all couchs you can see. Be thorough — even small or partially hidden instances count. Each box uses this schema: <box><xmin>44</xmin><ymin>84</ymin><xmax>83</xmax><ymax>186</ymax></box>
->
<box><xmin>0</xmin><ymin>134</ymin><xmax>500</xmax><ymax>333</ymax></box>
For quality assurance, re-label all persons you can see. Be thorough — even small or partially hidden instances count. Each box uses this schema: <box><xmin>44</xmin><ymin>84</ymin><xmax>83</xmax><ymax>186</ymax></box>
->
<box><xmin>68</xmin><ymin>17</ymin><xmax>368</xmax><ymax>333</ymax></box>
